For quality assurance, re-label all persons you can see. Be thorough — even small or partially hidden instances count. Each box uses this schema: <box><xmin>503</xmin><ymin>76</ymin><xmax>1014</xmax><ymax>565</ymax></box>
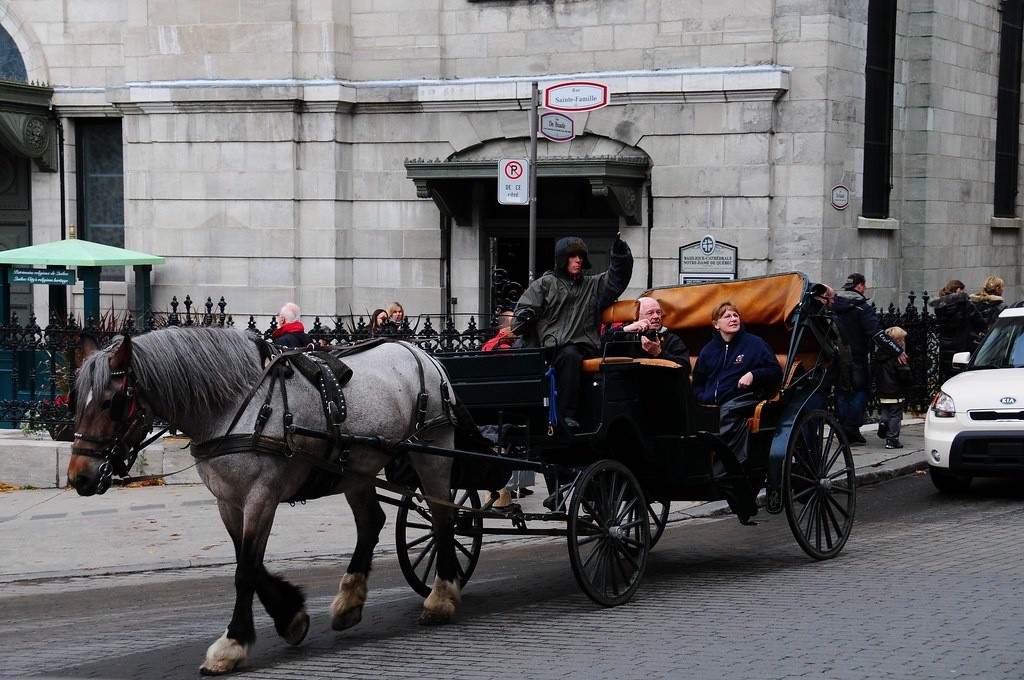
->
<box><xmin>387</xmin><ymin>302</ymin><xmax>404</xmax><ymax>330</ymax></box>
<box><xmin>313</xmin><ymin>326</ymin><xmax>331</xmax><ymax>345</ymax></box>
<box><xmin>930</xmin><ymin>281</ymin><xmax>987</xmax><ymax>384</ymax></box>
<box><xmin>835</xmin><ymin>273</ymin><xmax>908</xmax><ymax>446</ymax></box>
<box><xmin>876</xmin><ymin>326</ymin><xmax>911</xmax><ymax>449</ymax></box>
<box><xmin>513</xmin><ymin>231</ymin><xmax>634</xmax><ymax>426</ymax></box>
<box><xmin>793</xmin><ymin>282</ymin><xmax>842</xmax><ymax>462</ymax></box>
<box><xmin>365</xmin><ymin>308</ymin><xmax>388</xmax><ymax>331</ymax></box>
<box><xmin>273</xmin><ymin>303</ymin><xmax>312</xmax><ymax>346</ymax></box>
<box><xmin>481</xmin><ymin>305</ymin><xmax>534</xmax><ymax>511</ymax></box>
<box><xmin>602</xmin><ymin>297</ymin><xmax>692</xmax><ymax>373</ymax></box>
<box><xmin>691</xmin><ymin>301</ymin><xmax>783</xmax><ymax>476</ymax></box>
<box><xmin>972</xmin><ymin>276</ymin><xmax>1024</xmax><ymax>336</ymax></box>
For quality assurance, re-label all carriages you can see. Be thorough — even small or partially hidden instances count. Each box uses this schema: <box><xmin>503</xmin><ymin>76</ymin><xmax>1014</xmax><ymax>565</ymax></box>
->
<box><xmin>66</xmin><ymin>271</ymin><xmax>856</xmax><ymax>675</ymax></box>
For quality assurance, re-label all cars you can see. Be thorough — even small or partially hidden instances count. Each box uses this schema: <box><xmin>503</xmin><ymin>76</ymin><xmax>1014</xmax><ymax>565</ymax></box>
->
<box><xmin>923</xmin><ymin>305</ymin><xmax>1024</xmax><ymax>493</ymax></box>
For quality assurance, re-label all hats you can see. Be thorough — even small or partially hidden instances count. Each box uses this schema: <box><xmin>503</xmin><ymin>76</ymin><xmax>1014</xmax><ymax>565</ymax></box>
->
<box><xmin>842</xmin><ymin>273</ymin><xmax>865</xmax><ymax>288</ymax></box>
<box><xmin>556</xmin><ymin>239</ymin><xmax>592</xmax><ymax>273</ymax></box>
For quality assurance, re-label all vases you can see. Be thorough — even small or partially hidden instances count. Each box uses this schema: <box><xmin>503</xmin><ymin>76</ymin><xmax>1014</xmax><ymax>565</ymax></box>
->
<box><xmin>48</xmin><ymin>426</ymin><xmax>76</xmax><ymax>442</ymax></box>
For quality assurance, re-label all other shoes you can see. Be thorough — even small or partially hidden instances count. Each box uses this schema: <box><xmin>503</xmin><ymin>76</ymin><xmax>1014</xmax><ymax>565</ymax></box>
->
<box><xmin>510</xmin><ymin>488</ymin><xmax>533</xmax><ymax>497</ymax></box>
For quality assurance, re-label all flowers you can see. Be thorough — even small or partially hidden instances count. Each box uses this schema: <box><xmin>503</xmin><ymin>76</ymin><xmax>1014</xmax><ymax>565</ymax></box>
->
<box><xmin>19</xmin><ymin>396</ymin><xmax>75</xmax><ymax>440</ymax></box>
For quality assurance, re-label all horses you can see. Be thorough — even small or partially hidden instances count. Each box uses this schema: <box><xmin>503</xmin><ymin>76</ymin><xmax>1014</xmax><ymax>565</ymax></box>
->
<box><xmin>66</xmin><ymin>323</ymin><xmax>468</xmax><ymax>676</ymax></box>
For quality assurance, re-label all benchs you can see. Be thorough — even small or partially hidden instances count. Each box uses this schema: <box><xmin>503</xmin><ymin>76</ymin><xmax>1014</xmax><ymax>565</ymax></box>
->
<box><xmin>547</xmin><ymin>298</ymin><xmax>643</xmax><ymax>432</ymax></box>
<box><xmin>626</xmin><ymin>337</ymin><xmax>807</xmax><ymax>442</ymax></box>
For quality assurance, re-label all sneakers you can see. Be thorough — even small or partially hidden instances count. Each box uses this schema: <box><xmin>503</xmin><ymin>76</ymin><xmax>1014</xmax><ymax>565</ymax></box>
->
<box><xmin>885</xmin><ymin>439</ymin><xmax>905</xmax><ymax>448</ymax></box>
<box><xmin>564</xmin><ymin>417</ymin><xmax>580</xmax><ymax>429</ymax></box>
<box><xmin>845</xmin><ymin>428</ymin><xmax>868</xmax><ymax>446</ymax></box>
<box><xmin>877</xmin><ymin>423</ymin><xmax>888</xmax><ymax>438</ymax></box>
